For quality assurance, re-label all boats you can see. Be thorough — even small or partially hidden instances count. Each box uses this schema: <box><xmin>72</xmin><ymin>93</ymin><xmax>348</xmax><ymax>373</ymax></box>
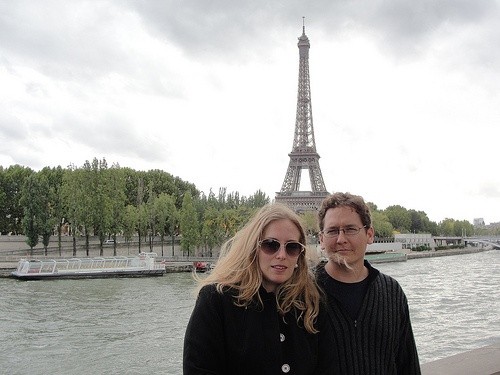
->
<box><xmin>9</xmin><ymin>253</ymin><xmax>167</xmax><ymax>279</ymax></box>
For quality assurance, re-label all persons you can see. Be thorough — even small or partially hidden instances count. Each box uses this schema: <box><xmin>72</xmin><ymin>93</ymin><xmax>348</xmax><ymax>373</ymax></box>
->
<box><xmin>308</xmin><ymin>192</ymin><xmax>422</xmax><ymax>375</ymax></box>
<box><xmin>183</xmin><ymin>205</ymin><xmax>334</xmax><ymax>375</ymax></box>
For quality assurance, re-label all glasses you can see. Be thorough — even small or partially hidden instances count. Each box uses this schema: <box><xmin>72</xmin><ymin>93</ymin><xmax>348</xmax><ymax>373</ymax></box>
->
<box><xmin>258</xmin><ymin>239</ymin><xmax>305</xmax><ymax>256</ymax></box>
<box><xmin>318</xmin><ymin>225</ymin><xmax>367</xmax><ymax>238</ymax></box>
